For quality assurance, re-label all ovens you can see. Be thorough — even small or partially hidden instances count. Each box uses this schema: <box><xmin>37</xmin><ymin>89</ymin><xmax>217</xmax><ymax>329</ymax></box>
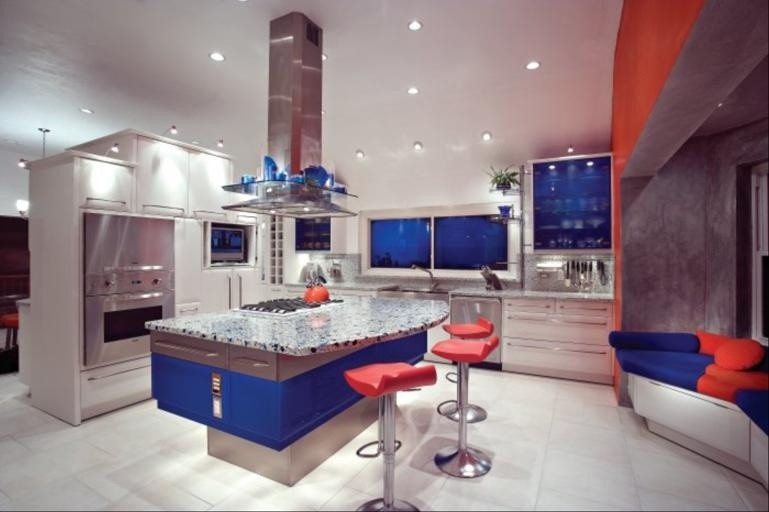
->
<box><xmin>84</xmin><ymin>290</ymin><xmax>175</xmax><ymax>365</ymax></box>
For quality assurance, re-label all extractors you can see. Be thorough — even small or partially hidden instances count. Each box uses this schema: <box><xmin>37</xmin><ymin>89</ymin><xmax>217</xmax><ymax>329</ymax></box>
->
<box><xmin>221</xmin><ymin>176</ymin><xmax>357</xmax><ymax>221</ymax></box>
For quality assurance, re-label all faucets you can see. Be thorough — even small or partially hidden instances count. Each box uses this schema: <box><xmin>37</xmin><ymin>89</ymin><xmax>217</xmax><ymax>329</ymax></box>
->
<box><xmin>413</xmin><ymin>264</ymin><xmax>434</xmax><ymax>285</ymax></box>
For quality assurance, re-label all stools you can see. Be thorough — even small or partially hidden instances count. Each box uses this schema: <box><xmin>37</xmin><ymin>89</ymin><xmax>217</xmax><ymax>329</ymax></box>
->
<box><xmin>431</xmin><ymin>316</ymin><xmax>503</xmax><ymax>482</ymax></box>
<box><xmin>343</xmin><ymin>360</ymin><xmax>440</xmax><ymax>512</ymax></box>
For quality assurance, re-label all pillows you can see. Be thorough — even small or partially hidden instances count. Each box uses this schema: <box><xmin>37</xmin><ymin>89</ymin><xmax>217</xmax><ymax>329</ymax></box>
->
<box><xmin>607</xmin><ymin>328</ymin><xmax>765</xmax><ymax>371</ymax></box>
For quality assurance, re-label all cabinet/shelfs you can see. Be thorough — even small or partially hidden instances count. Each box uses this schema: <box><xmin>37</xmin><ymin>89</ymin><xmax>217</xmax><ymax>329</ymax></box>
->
<box><xmin>73</xmin><ymin>134</ymin><xmax>233</xmax><ymax>224</ymax></box>
<box><xmin>501</xmin><ymin>299</ymin><xmax>614</xmax><ymax>386</ymax></box>
<box><xmin>78</xmin><ymin>355</ymin><xmax>150</xmax><ymax>410</ymax></box>
<box><xmin>234</xmin><ymin>193</ymin><xmax>257</xmax><ymax>224</ymax></box>
<box><xmin>27</xmin><ymin>157</ymin><xmax>134</xmax><ymax>213</ymax></box>
<box><xmin>174</xmin><ymin>301</ymin><xmax>202</xmax><ymax>318</ymax></box>
<box><xmin>201</xmin><ymin>265</ymin><xmax>261</xmax><ymax>313</ymax></box>
<box><xmin>487</xmin><ymin>188</ymin><xmax>523</xmax><ymax>266</ymax></box>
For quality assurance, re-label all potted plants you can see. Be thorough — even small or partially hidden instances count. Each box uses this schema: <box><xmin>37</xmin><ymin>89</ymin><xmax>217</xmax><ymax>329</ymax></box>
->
<box><xmin>484</xmin><ymin>162</ymin><xmax>520</xmax><ymax>191</ymax></box>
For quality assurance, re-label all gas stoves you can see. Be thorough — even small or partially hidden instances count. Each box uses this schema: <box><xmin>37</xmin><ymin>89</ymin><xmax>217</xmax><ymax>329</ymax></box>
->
<box><xmin>232</xmin><ymin>297</ymin><xmax>345</xmax><ymax>318</ymax></box>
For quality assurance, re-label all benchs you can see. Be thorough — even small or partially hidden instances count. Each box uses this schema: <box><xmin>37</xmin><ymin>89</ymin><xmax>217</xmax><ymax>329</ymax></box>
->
<box><xmin>609</xmin><ymin>325</ymin><xmax>769</xmax><ymax>503</ymax></box>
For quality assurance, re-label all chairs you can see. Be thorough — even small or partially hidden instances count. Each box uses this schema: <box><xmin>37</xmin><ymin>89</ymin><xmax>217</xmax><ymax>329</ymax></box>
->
<box><xmin>0</xmin><ymin>295</ymin><xmax>22</xmax><ymax>349</ymax></box>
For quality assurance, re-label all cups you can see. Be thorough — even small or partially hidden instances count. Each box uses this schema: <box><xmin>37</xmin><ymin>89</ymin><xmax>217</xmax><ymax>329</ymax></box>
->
<box><xmin>537</xmin><ymin>196</ymin><xmax>609</xmax><ymax>248</ymax></box>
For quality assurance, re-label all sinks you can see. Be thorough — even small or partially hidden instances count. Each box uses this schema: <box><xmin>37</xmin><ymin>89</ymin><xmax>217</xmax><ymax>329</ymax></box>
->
<box><xmin>378</xmin><ymin>284</ymin><xmax>455</xmax><ymax>295</ymax></box>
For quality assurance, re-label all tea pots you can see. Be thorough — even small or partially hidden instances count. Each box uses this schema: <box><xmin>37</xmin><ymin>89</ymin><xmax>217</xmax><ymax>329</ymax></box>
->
<box><xmin>304</xmin><ymin>277</ymin><xmax>329</xmax><ymax>302</ymax></box>
